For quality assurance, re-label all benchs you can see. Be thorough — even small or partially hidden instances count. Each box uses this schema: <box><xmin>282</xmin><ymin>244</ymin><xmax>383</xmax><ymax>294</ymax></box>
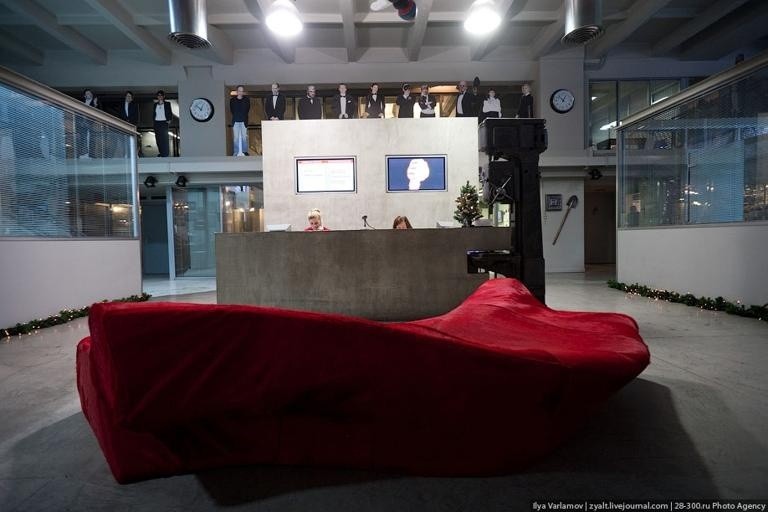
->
<box><xmin>77</xmin><ymin>275</ymin><xmax>650</xmax><ymax>508</ymax></box>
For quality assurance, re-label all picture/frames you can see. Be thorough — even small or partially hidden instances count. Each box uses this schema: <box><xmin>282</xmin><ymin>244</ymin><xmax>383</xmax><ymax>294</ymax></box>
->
<box><xmin>545</xmin><ymin>195</ymin><xmax>562</xmax><ymax>211</ymax></box>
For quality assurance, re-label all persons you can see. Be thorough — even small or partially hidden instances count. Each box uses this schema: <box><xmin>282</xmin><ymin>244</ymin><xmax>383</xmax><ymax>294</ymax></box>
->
<box><xmin>74</xmin><ymin>88</ymin><xmax>99</xmax><ymax>159</ymax></box>
<box><xmin>303</xmin><ymin>209</ymin><xmax>328</xmax><ymax>231</ymax></box>
<box><xmin>417</xmin><ymin>84</ymin><xmax>436</xmax><ymax>117</ymax></box>
<box><xmin>120</xmin><ymin>91</ymin><xmax>139</xmax><ymax>157</ymax></box>
<box><xmin>230</xmin><ymin>85</ymin><xmax>250</xmax><ymax>156</ymax></box>
<box><xmin>396</xmin><ymin>83</ymin><xmax>416</xmax><ymax>118</ymax></box>
<box><xmin>298</xmin><ymin>85</ymin><xmax>322</xmax><ymax>119</ymax></box>
<box><xmin>514</xmin><ymin>84</ymin><xmax>534</xmax><ymax>118</ymax></box>
<box><xmin>263</xmin><ymin>82</ymin><xmax>286</xmax><ymax>121</ymax></box>
<box><xmin>480</xmin><ymin>88</ymin><xmax>502</xmax><ymax>121</ymax></box>
<box><xmin>393</xmin><ymin>215</ymin><xmax>412</xmax><ymax>229</ymax></box>
<box><xmin>627</xmin><ymin>206</ymin><xmax>639</xmax><ymax>227</ymax></box>
<box><xmin>364</xmin><ymin>83</ymin><xmax>385</xmax><ymax>118</ymax></box>
<box><xmin>455</xmin><ymin>81</ymin><xmax>475</xmax><ymax>117</ymax></box>
<box><xmin>331</xmin><ymin>83</ymin><xmax>355</xmax><ymax>119</ymax></box>
<box><xmin>152</xmin><ymin>90</ymin><xmax>172</xmax><ymax>157</ymax></box>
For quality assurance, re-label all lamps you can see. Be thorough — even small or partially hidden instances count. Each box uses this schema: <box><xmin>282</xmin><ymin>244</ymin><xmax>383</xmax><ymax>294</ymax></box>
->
<box><xmin>590</xmin><ymin>169</ymin><xmax>603</xmax><ymax>180</ymax></box>
<box><xmin>460</xmin><ymin>1</ymin><xmax>504</xmax><ymax>37</ymax></box>
<box><xmin>144</xmin><ymin>176</ymin><xmax>157</xmax><ymax>188</ymax></box>
<box><xmin>175</xmin><ymin>176</ymin><xmax>187</xmax><ymax>187</ymax></box>
<box><xmin>265</xmin><ymin>0</ymin><xmax>305</xmax><ymax>41</ymax></box>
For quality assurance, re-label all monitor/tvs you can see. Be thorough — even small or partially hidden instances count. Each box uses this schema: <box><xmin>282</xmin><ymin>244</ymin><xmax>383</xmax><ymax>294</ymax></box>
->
<box><xmin>438</xmin><ymin>222</ymin><xmax>455</xmax><ymax>227</ymax></box>
<box><xmin>267</xmin><ymin>224</ymin><xmax>290</xmax><ymax>232</ymax></box>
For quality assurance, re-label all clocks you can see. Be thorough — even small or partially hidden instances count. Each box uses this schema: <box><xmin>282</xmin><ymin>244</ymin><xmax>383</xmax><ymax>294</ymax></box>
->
<box><xmin>190</xmin><ymin>98</ymin><xmax>214</xmax><ymax>122</ymax></box>
<box><xmin>550</xmin><ymin>89</ymin><xmax>575</xmax><ymax>114</ymax></box>
